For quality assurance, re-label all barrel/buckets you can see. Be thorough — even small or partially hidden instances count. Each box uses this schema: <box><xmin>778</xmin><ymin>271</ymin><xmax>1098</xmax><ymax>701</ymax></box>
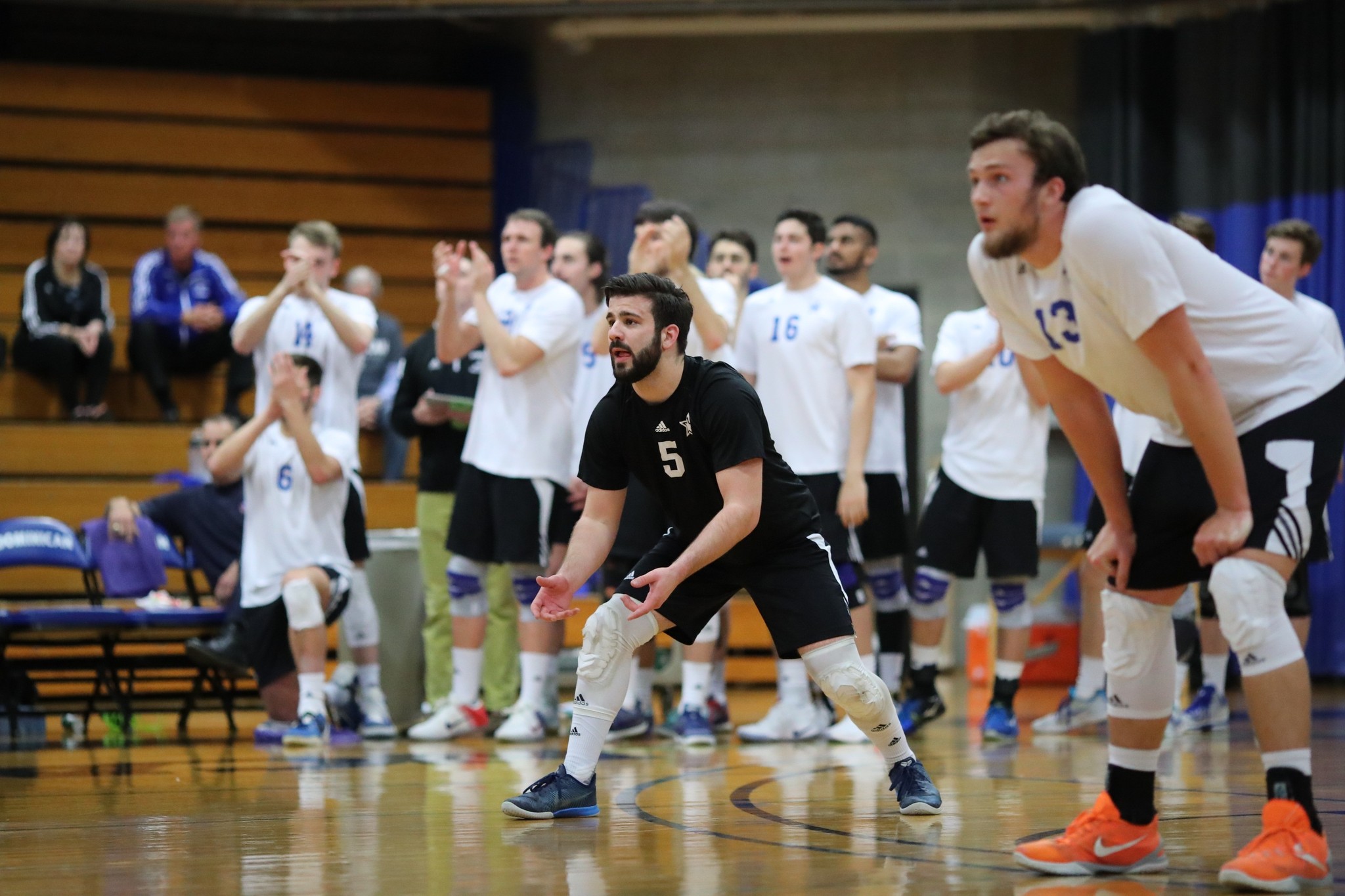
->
<box><xmin>364</xmin><ymin>531</ymin><xmax>427</xmax><ymax>729</ymax></box>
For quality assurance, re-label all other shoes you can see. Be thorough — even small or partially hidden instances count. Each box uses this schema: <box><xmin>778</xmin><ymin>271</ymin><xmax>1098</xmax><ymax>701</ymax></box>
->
<box><xmin>657</xmin><ymin>700</ymin><xmax>733</xmax><ymax>737</ymax></box>
<box><xmin>825</xmin><ymin>716</ymin><xmax>870</xmax><ymax>741</ymax></box>
<box><xmin>252</xmin><ymin>719</ymin><xmax>293</xmax><ymax>742</ymax></box>
<box><xmin>495</xmin><ymin>703</ymin><xmax>559</xmax><ymax>740</ymax></box>
<box><xmin>283</xmin><ymin>713</ymin><xmax>329</xmax><ymax>746</ymax></box>
<box><xmin>357</xmin><ymin>702</ymin><xmax>398</xmax><ymax>737</ymax></box>
<box><xmin>982</xmin><ymin>704</ymin><xmax>1018</xmax><ymax>740</ymax></box>
<box><xmin>898</xmin><ymin>689</ymin><xmax>945</xmax><ymax>733</ymax></box>
<box><xmin>738</xmin><ymin>701</ymin><xmax>818</xmax><ymax>740</ymax></box>
<box><xmin>1182</xmin><ymin>685</ymin><xmax>1231</xmax><ymax>733</ymax></box>
<box><xmin>1031</xmin><ymin>688</ymin><xmax>1108</xmax><ymax>733</ymax></box>
<box><xmin>672</xmin><ymin>704</ymin><xmax>719</xmax><ymax>747</ymax></box>
<box><xmin>607</xmin><ymin>700</ymin><xmax>651</xmax><ymax>742</ymax></box>
<box><xmin>408</xmin><ymin>703</ymin><xmax>488</xmax><ymax>740</ymax></box>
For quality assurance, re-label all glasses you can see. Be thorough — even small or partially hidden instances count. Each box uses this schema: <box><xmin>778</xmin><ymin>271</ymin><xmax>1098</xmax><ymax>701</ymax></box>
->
<box><xmin>201</xmin><ymin>439</ymin><xmax>222</xmax><ymax>447</ymax></box>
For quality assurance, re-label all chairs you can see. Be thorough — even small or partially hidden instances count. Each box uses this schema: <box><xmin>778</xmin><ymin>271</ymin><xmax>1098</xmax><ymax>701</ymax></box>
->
<box><xmin>0</xmin><ymin>515</ymin><xmax>254</xmax><ymax>749</ymax></box>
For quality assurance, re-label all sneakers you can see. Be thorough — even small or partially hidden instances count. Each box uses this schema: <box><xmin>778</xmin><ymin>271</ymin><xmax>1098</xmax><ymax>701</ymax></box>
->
<box><xmin>501</xmin><ymin>764</ymin><xmax>598</xmax><ymax>820</ymax></box>
<box><xmin>887</xmin><ymin>757</ymin><xmax>942</xmax><ymax>816</ymax></box>
<box><xmin>1218</xmin><ymin>794</ymin><xmax>1333</xmax><ymax>893</ymax></box>
<box><xmin>1011</xmin><ymin>790</ymin><xmax>1171</xmax><ymax>875</ymax></box>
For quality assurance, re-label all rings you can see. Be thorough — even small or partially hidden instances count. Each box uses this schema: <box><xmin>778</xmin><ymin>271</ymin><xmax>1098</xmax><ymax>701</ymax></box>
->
<box><xmin>113</xmin><ymin>524</ymin><xmax>119</xmax><ymax>533</ymax></box>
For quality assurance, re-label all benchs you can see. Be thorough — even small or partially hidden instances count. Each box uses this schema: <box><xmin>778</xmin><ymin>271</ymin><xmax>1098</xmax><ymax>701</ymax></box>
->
<box><xmin>0</xmin><ymin>62</ymin><xmax>778</xmax><ymax>695</ymax></box>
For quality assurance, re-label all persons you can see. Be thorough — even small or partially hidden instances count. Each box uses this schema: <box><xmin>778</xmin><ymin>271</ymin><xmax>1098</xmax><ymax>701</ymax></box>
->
<box><xmin>122</xmin><ymin>205</ymin><xmax>255</xmax><ymax>428</ymax></box>
<box><xmin>207</xmin><ymin>196</ymin><xmax>920</xmax><ymax>748</ymax></box>
<box><xmin>1030</xmin><ymin>214</ymin><xmax>1217</xmax><ymax>740</ymax></box>
<box><xmin>964</xmin><ymin>109</ymin><xmax>1345</xmax><ymax>896</ymax></box>
<box><xmin>501</xmin><ymin>268</ymin><xmax>944</xmax><ymax>818</ymax></box>
<box><xmin>10</xmin><ymin>210</ymin><xmax>115</xmax><ymax>423</ymax></box>
<box><xmin>107</xmin><ymin>415</ymin><xmax>302</xmax><ymax>744</ymax></box>
<box><xmin>1164</xmin><ymin>219</ymin><xmax>1345</xmax><ymax>736</ymax></box>
<box><xmin>897</xmin><ymin>302</ymin><xmax>1052</xmax><ymax>739</ymax></box>
